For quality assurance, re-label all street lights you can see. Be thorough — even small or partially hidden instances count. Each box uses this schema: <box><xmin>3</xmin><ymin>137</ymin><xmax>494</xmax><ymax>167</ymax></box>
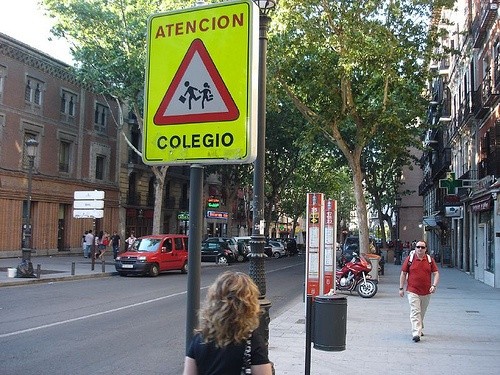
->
<box><xmin>14</xmin><ymin>137</ymin><xmax>39</xmax><ymax>278</ymax></box>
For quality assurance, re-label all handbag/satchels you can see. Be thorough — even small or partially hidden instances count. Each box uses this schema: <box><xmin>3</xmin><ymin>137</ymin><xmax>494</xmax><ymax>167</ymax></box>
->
<box><xmin>103</xmin><ymin>237</ymin><xmax>110</xmax><ymax>247</ymax></box>
<box><xmin>241</xmin><ymin>330</ymin><xmax>275</xmax><ymax>374</ymax></box>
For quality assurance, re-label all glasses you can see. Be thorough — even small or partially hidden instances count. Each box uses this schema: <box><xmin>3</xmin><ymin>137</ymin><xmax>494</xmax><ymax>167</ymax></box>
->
<box><xmin>415</xmin><ymin>245</ymin><xmax>425</xmax><ymax>250</ymax></box>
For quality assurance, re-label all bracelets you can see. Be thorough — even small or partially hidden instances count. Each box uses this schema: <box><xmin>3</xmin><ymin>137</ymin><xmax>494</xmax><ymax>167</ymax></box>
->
<box><xmin>432</xmin><ymin>284</ymin><xmax>437</xmax><ymax>289</ymax></box>
<box><xmin>400</xmin><ymin>288</ymin><xmax>403</xmax><ymax>290</ymax></box>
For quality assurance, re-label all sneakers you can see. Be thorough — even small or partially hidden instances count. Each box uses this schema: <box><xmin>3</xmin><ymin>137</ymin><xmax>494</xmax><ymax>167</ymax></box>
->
<box><xmin>412</xmin><ymin>335</ymin><xmax>420</xmax><ymax>343</ymax></box>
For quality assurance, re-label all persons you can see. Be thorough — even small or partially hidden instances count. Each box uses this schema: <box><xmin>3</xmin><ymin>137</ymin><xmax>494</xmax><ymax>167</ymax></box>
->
<box><xmin>81</xmin><ymin>230</ymin><xmax>138</xmax><ymax>259</ymax></box>
<box><xmin>367</xmin><ymin>239</ymin><xmax>416</xmax><ymax>265</ymax></box>
<box><xmin>182</xmin><ymin>270</ymin><xmax>272</xmax><ymax>375</ymax></box>
<box><xmin>400</xmin><ymin>241</ymin><xmax>440</xmax><ymax>342</ymax></box>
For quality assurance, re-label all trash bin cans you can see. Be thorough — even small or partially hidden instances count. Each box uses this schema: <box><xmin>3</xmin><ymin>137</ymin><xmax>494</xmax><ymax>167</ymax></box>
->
<box><xmin>314</xmin><ymin>291</ymin><xmax>347</xmax><ymax>352</ymax></box>
<box><xmin>365</xmin><ymin>254</ymin><xmax>382</xmax><ymax>283</ymax></box>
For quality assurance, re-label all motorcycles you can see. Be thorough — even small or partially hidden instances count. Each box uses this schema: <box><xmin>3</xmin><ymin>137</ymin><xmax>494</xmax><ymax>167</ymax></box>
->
<box><xmin>336</xmin><ymin>252</ymin><xmax>377</xmax><ymax>298</ymax></box>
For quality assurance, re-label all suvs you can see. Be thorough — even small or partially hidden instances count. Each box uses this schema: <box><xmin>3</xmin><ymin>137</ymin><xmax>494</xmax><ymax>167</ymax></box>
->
<box><xmin>340</xmin><ymin>237</ymin><xmax>377</xmax><ymax>261</ymax></box>
<box><xmin>115</xmin><ymin>234</ymin><xmax>189</xmax><ymax>277</ymax></box>
<box><xmin>269</xmin><ymin>241</ymin><xmax>285</xmax><ymax>259</ymax></box>
<box><xmin>201</xmin><ymin>242</ymin><xmax>234</xmax><ymax>263</ymax></box>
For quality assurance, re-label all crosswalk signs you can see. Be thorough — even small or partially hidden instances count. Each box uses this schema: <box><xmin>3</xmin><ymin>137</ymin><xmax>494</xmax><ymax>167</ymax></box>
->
<box><xmin>141</xmin><ymin>0</ymin><xmax>259</xmax><ymax>166</ymax></box>
